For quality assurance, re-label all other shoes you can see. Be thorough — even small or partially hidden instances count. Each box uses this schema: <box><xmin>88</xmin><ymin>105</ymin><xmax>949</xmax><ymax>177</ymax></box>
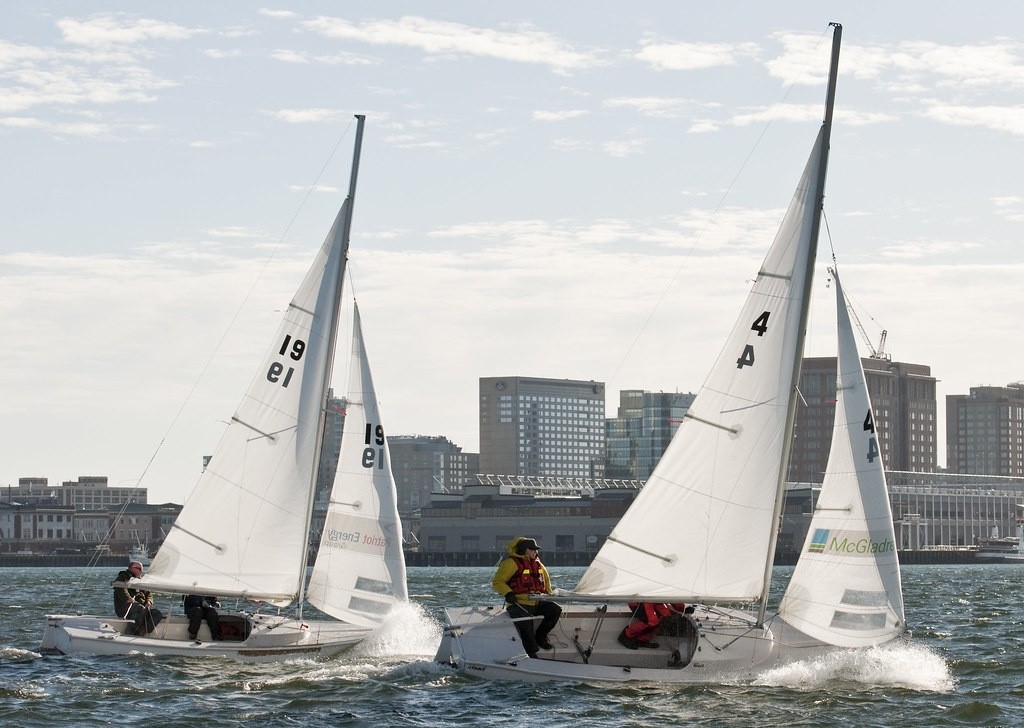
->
<box><xmin>189</xmin><ymin>635</ymin><xmax>196</xmax><ymax>640</ymax></box>
<box><xmin>637</xmin><ymin>640</ymin><xmax>658</xmax><ymax>648</ymax></box>
<box><xmin>618</xmin><ymin>632</ymin><xmax>637</xmax><ymax>649</ymax></box>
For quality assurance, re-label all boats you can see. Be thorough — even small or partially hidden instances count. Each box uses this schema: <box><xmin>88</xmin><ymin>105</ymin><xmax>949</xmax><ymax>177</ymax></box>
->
<box><xmin>128</xmin><ymin>547</ymin><xmax>150</xmax><ymax>567</ymax></box>
<box><xmin>974</xmin><ymin>536</ymin><xmax>1023</xmax><ymax>563</ymax></box>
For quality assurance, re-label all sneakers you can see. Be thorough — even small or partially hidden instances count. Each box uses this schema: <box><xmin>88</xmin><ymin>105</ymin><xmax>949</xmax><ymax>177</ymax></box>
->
<box><xmin>536</xmin><ymin>635</ymin><xmax>552</xmax><ymax>649</ymax></box>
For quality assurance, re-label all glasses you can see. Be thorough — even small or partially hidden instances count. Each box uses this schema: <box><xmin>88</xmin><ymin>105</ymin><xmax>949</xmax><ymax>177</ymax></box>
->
<box><xmin>134</xmin><ymin>566</ymin><xmax>142</xmax><ymax>571</ymax></box>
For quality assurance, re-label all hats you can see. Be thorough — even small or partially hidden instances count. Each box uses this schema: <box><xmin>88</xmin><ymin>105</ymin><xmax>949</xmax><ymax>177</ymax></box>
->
<box><xmin>518</xmin><ymin>538</ymin><xmax>540</xmax><ymax>550</ymax></box>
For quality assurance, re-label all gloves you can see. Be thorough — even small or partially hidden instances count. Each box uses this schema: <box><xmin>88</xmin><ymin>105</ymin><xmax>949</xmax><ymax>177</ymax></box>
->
<box><xmin>505</xmin><ymin>592</ymin><xmax>517</xmax><ymax>605</ymax></box>
<box><xmin>685</xmin><ymin>606</ymin><xmax>694</xmax><ymax>614</ymax></box>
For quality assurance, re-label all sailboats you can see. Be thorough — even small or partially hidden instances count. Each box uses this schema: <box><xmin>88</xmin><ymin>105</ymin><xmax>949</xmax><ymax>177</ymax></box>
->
<box><xmin>37</xmin><ymin>111</ymin><xmax>443</xmax><ymax>672</ymax></box>
<box><xmin>434</xmin><ymin>13</ymin><xmax>950</xmax><ymax>696</ymax></box>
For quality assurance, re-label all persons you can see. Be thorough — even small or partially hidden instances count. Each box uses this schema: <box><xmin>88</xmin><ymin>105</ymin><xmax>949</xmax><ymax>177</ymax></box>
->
<box><xmin>492</xmin><ymin>537</ymin><xmax>562</xmax><ymax>659</ymax></box>
<box><xmin>112</xmin><ymin>560</ymin><xmax>162</xmax><ymax>636</ymax></box>
<box><xmin>180</xmin><ymin>593</ymin><xmax>224</xmax><ymax>642</ymax></box>
<box><xmin>618</xmin><ymin>600</ymin><xmax>685</xmax><ymax>651</ymax></box>
